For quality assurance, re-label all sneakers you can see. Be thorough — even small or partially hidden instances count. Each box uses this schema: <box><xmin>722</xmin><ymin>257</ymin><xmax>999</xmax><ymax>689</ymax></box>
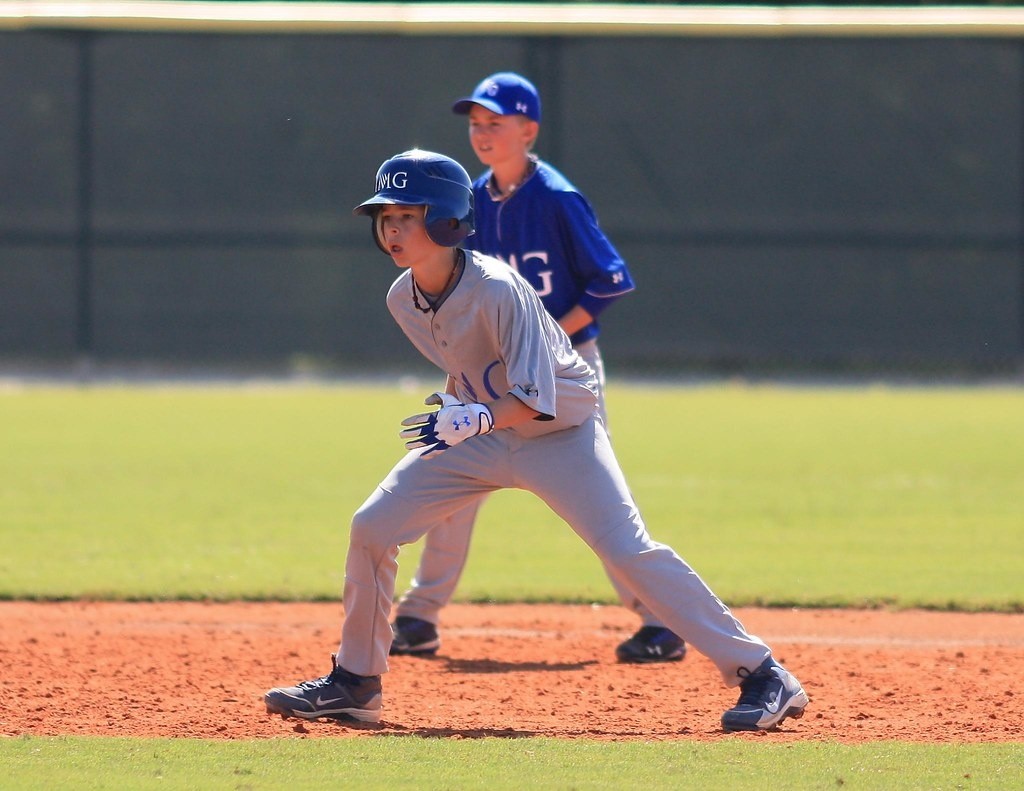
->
<box><xmin>389</xmin><ymin>616</ymin><xmax>440</xmax><ymax>653</ymax></box>
<box><xmin>615</xmin><ymin>625</ymin><xmax>686</xmax><ymax>663</ymax></box>
<box><xmin>264</xmin><ymin>653</ymin><xmax>382</xmax><ymax>723</ymax></box>
<box><xmin>721</xmin><ymin>656</ymin><xmax>809</xmax><ymax>732</ymax></box>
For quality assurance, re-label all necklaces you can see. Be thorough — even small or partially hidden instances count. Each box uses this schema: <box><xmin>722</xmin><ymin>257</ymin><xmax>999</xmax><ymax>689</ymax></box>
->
<box><xmin>411</xmin><ymin>248</ymin><xmax>461</xmax><ymax>313</ymax></box>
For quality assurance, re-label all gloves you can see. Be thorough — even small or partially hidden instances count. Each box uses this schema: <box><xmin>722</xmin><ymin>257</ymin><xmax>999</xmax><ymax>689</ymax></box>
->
<box><xmin>398</xmin><ymin>393</ymin><xmax>494</xmax><ymax>460</ymax></box>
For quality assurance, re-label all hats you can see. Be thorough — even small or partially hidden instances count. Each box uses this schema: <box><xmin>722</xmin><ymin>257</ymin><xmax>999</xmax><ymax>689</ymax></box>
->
<box><xmin>452</xmin><ymin>72</ymin><xmax>541</xmax><ymax>124</ymax></box>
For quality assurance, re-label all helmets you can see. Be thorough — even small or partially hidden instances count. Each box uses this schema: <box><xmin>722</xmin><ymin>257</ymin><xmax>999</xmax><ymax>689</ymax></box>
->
<box><xmin>352</xmin><ymin>150</ymin><xmax>475</xmax><ymax>255</ymax></box>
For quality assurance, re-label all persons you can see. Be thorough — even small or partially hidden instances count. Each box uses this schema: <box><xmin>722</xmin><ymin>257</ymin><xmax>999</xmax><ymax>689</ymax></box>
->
<box><xmin>264</xmin><ymin>149</ymin><xmax>809</xmax><ymax>731</ymax></box>
<box><xmin>390</xmin><ymin>73</ymin><xmax>686</xmax><ymax>663</ymax></box>
